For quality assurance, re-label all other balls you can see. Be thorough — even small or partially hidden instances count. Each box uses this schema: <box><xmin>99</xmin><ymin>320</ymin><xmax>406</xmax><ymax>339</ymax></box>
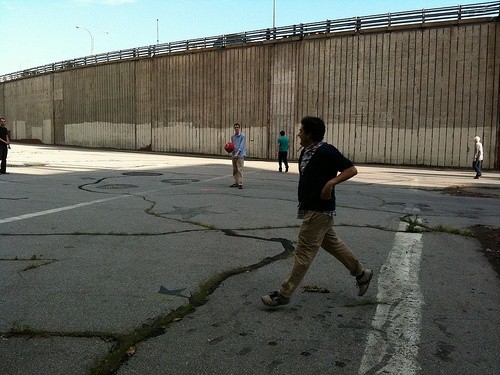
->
<box><xmin>225</xmin><ymin>143</ymin><xmax>234</xmax><ymax>153</ymax></box>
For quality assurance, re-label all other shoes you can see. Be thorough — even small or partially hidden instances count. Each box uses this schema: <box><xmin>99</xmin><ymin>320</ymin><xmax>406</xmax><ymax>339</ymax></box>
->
<box><xmin>285</xmin><ymin>166</ymin><xmax>289</xmax><ymax>172</ymax></box>
<box><xmin>0</xmin><ymin>169</ymin><xmax>9</xmax><ymax>175</ymax></box>
<box><xmin>474</xmin><ymin>174</ymin><xmax>482</xmax><ymax>179</ymax></box>
<box><xmin>279</xmin><ymin>168</ymin><xmax>282</xmax><ymax>172</ymax></box>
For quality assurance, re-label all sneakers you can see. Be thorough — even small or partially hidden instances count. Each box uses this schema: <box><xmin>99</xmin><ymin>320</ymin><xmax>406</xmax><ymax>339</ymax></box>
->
<box><xmin>239</xmin><ymin>185</ymin><xmax>243</xmax><ymax>189</ymax></box>
<box><xmin>355</xmin><ymin>268</ymin><xmax>373</xmax><ymax>296</ymax></box>
<box><xmin>260</xmin><ymin>290</ymin><xmax>291</xmax><ymax>307</ymax></box>
<box><xmin>230</xmin><ymin>183</ymin><xmax>239</xmax><ymax>187</ymax></box>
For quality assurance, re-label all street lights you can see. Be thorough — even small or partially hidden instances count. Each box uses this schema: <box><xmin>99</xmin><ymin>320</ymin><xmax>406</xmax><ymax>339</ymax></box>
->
<box><xmin>75</xmin><ymin>25</ymin><xmax>109</xmax><ymax>56</ymax></box>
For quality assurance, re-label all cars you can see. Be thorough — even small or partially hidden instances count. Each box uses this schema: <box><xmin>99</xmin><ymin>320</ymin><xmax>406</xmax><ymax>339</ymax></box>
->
<box><xmin>213</xmin><ymin>33</ymin><xmax>254</xmax><ymax>47</ymax></box>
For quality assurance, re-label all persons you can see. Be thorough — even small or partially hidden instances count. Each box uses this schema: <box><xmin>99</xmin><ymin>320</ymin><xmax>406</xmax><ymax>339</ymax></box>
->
<box><xmin>261</xmin><ymin>116</ymin><xmax>374</xmax><ymax>306</ymax></box>
<box><xmin>277</xmin><ymin>131</ymin><xmax>289</xmax><ymax>172</ymax></box>
<box><xmin>0</xmin><ymin>118</ymin><xmax>11</xmax><ymax>175</ymax></box>
<box><xmin>473</xmin><ymin>136</ymin><xmax>483</xmax><ymax>179</ymax></box>
<box><xmin>224</xmin><ymin>123</ymin><xmax>246</xmax><ymax>189</ymax></box>
